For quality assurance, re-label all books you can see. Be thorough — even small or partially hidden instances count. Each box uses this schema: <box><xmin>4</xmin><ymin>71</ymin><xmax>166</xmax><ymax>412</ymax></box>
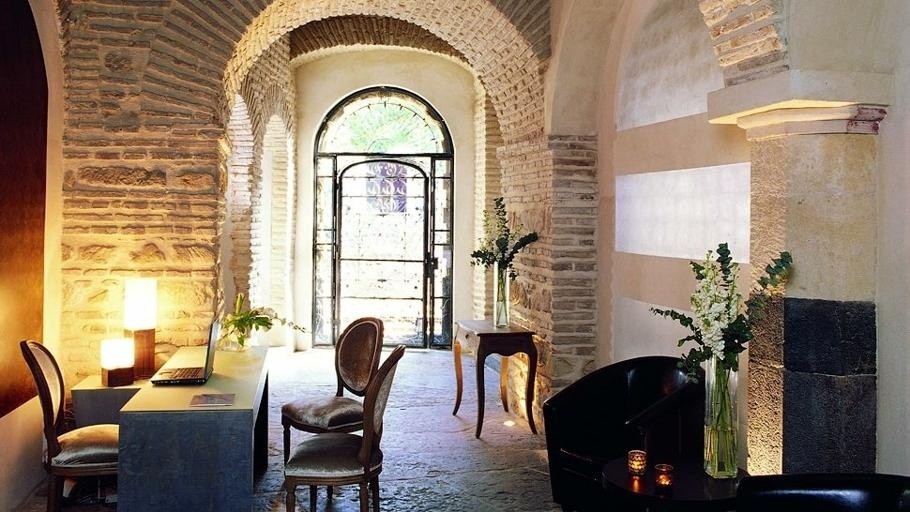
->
<box><xmin>190</xmin><ymin>393</ymin><xmax>237</xmax><ymax>405</ymax></box>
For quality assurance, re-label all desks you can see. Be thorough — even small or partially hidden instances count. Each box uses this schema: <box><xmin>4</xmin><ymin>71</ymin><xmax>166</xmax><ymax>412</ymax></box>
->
<box><xmin>452</xmin><ymin>319</ymin><xmax>537</xmax><ymax>438</ymax></box>
<box><xmin>116</xmin><ymin>345</ymin><xmax>269</xmax><ymax>511</ymax></box>
<box><xmin>602</xmin><ymin>453</ymin><xmax>750</xmax><ymax>511</ymax></box>
<box><xmin>69</xmin><ymin>374</ymin><xmax>151</xmax><ymax>425</ymax></box>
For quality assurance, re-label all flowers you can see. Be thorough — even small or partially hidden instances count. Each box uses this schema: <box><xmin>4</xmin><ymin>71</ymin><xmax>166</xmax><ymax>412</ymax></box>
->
<box><xmin>221</xmin><ymin>292</ymin><xmax>305</xmax><ymax>348</ymax></box>
<box><xmin>648</xmin><ymin>242</ymin><xmax>794</xmax><ymax>476</ymax></box>
<box><xmin>469</xmin><ymin>196</ymin><xmax>538</xmax><ymax>323</ymax></box>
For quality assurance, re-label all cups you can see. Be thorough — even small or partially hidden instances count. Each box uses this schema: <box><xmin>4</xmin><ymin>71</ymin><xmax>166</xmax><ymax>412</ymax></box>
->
<box><xmin>628</xmin><ymin>450</ymin><xmax>647</xmax><ymax>475</ymax></box>
<box><xmin>654</xmin><ymin>464</ymin><xmax>674</xmax><ymax>489</ymax></box>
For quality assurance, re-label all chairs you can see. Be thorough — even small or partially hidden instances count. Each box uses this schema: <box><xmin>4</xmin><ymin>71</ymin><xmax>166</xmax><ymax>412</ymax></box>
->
<box><xmin>283</xmin><ymin>344</ymin><xmax>406</xmax><ymax>512</ymax></box>
<box><xmin>735</xmin><ymin>473</ymin><xmax>910</xmax><ymax>512</ymax></box>
<box><xmin>542</xmin><ymin>355</ymin><xmax>706</xmax><ymax>511</ymax></box>
<box><xmin>281</xmin><ymin>317</ymin><xmax>384</xmax><ymax>467</ymax></box>
<box><xmin>21</xmin><ymin>342</ymin><xmax>119</xmax><ymax>512</ymax></box>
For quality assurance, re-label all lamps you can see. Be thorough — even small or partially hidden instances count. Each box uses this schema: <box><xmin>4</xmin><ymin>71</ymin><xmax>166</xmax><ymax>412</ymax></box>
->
<box><xmin>123</xmin><ymin>277</ymin><xmax>158</xmax><ymax>379</ymax></box>
<box><xmin>100</xmin><ymin>338</ymin><xmax>135</xmax><ymax>387</ymax></box>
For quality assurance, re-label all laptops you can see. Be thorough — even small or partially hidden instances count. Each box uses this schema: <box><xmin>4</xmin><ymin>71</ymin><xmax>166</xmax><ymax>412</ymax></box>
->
<box><xmin>150</xmin><ymin>317</ymin><xmax>220</xmax><ymax>385</ymax></box>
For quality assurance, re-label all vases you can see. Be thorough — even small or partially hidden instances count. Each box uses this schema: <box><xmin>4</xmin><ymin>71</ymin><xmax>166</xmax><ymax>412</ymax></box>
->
<box><xmin>227</xmin><ymin>329</ymin><xmax>245</xmax><ymax>351</ymax></box>
<box><xmin>703</xmin><ymin>355</ymin><xmax>738</xmax><ymax>480</ymax></box>
<box><xmin>493</xmin><ymin>266</ymin><xmax>510</xmax><ymax>329</ymax></box>
